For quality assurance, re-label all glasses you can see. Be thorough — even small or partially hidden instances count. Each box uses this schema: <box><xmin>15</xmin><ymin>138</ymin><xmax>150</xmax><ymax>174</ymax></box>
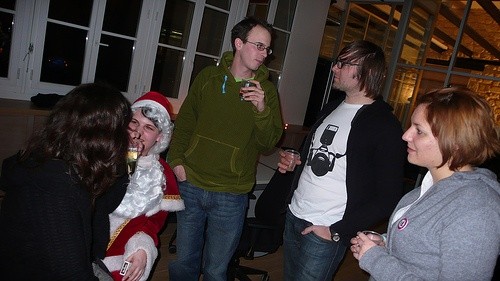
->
<box><xmin>246</xmin><ymin>40</ymin><xmax>273</xmax><ymax>55</ymax></box>
<box><xmin>333</xmin><ymin>59</ymin><xmax>359</xmax><ymax>69</ymax></box>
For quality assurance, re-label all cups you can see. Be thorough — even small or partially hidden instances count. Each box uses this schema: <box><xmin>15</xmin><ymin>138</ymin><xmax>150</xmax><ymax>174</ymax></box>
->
<box><xmin>284</xmin><ymin>150</ymin><xmax>300</xmax><ymax>171</ymax></box>
<box><xmin>240</xmin><ymin>80</ymin><xmax>256</xmax><ymax>102</ymax></box>
<box><xmin>363</xmin><ymin>231</ymin><xmax>384</xmax><ymax>245</ymax></box>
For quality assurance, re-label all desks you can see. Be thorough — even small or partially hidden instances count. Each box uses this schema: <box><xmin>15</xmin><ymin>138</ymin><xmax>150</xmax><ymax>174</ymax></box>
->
<box><xmin>201</xmin><ymin>147</ymin><xmax>292</xmax><ymax>281</ymax></box>
<box><xmin>0</xmin><ymin>97</ymin><xmax>54</xmax><ymax>160</ymax></box>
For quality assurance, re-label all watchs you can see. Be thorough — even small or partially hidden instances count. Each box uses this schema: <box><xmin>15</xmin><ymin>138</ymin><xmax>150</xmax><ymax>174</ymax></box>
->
<box><xmin>330</xmin><ymin>227</ymin><xmax>340</xmax><ymax>242</ymax></box>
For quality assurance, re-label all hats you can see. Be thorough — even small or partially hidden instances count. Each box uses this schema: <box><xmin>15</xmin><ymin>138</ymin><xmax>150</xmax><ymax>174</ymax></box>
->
<box><xmin>130</xmin><ymin>91</ymin><xmax>177</xmax><ymax>154</ymax></box>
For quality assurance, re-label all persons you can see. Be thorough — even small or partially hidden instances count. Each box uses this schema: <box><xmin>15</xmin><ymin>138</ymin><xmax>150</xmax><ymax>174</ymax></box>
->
<box><xmin>94</xmin><ymin>92</ymin><xmax>185</xmax><ymax>281</ymax></box>
<box><xmin>0</xmin><ymin>83</ymin><xmax>132</xmax><ymax>281</ymax></box>
<box><xmin>166</xmin><ymin>17</ymin><xmax>283</xmax><ymax>281</ymax></box>
<box><xmin>350</xmin><ymin>85</ymin><xmax>500</xmax><ymax>281</ymax></box>
<box><xmin>277</xmin><ymin>40</ymin><xmax>404</xmax><ymax>281</ymax></box>
